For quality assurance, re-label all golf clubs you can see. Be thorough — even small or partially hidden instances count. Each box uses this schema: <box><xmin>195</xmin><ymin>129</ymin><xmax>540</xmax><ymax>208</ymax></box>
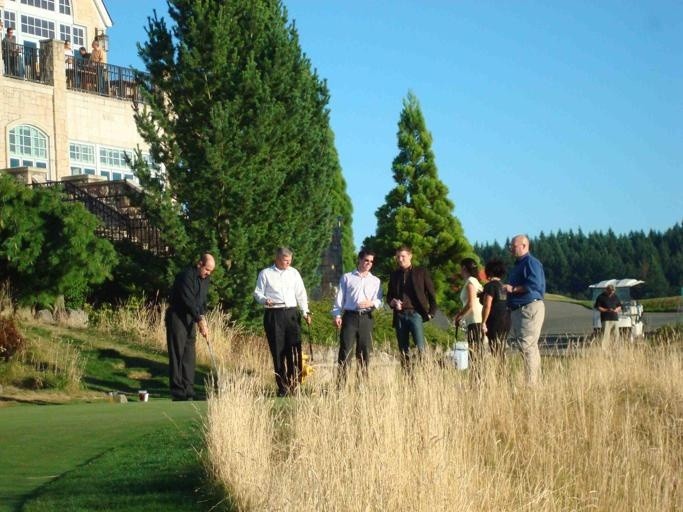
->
<box><xmin>306</xmin><ymin>312</ymin><xmax>314</xmax><ymax>361</ymax></box>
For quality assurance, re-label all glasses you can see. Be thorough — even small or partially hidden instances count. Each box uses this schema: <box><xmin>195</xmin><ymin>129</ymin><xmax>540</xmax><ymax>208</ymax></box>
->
<box><xmin>363</xmin><ymin>259</ymin><xmax>373</xmax><ymax>263</ymax></box>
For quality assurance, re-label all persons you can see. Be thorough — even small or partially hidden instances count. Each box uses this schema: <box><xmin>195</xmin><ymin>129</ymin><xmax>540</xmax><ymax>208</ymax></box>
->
<box><xmin>64</xmin><ymin>40</ymin><xmax>76</xmax><ymax>88</ymax></box>
<box><xmin>253</xmin><ymin>247</ymin><xmax>311</xmax><ymax>398</ymax></box>
<box><xmin>386</xmin><ymin>245</ymin><xmax>436</xmax><ymax>370</ymax></box>
<box><xmin>165</xmin><ymin>253</ymin><xmax>216</xmax><ymax>401</ymax></box>
<box><xmin>454</xmin><ymin>256</ymin><xmax>484</xmax><ymax>350</ymax></box>
<box><xmin>479</xmin><ymin>259</ymin><xmax>512</xmax><ymax>364</ymax></box>
<box><xmin>76</xmin><ymin>47</ymin><xmax>91</xmax><ymax>90</ymax></box>
<box><xmin>0</xmin><ymin>20</ymin><xmax>25</xmax><ymax>79</ymax></box>
<box><xmin>502</xmin><ymin>235</ymin><xmax>546</xmax><ymax>389</ymax></box>
<box><xmin>330</xmin><ymin>250</ymin><xmax>385</xmax><ymax>394</ymax></box>
<box><xmin>593</xmin><ymin>285</ymin><xmax>623</xmax><ymax>349</ymax></box>
<box><xmin>90</xmin><ymin>40</ymin><xmax>106</xmax><ymax>96</ymax></box>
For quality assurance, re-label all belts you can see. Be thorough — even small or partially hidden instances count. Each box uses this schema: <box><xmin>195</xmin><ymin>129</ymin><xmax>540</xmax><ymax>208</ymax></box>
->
<box><xmin>347</xmin><ymin>310</ymin><xmax>365</xmax><ymax>316</ymax></box>
<box><xmin>512</xmin><ymin>299</ymin><xmax>539</xmax><ymax>311</ymax></box>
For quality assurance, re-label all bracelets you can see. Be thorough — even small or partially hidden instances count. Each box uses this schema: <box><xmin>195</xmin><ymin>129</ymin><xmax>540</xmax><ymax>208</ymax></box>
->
<box><xmin>512</xmin><ymin>284</ymin><xmax>516</xmax><ymax>293</ymax></box>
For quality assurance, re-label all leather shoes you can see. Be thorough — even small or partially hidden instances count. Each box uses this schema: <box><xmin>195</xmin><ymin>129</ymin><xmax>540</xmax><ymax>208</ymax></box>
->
<box><xmin>171</xmin><ymin>395</ymin><xmax>206</xmax><ymax>400</ymax></box>
